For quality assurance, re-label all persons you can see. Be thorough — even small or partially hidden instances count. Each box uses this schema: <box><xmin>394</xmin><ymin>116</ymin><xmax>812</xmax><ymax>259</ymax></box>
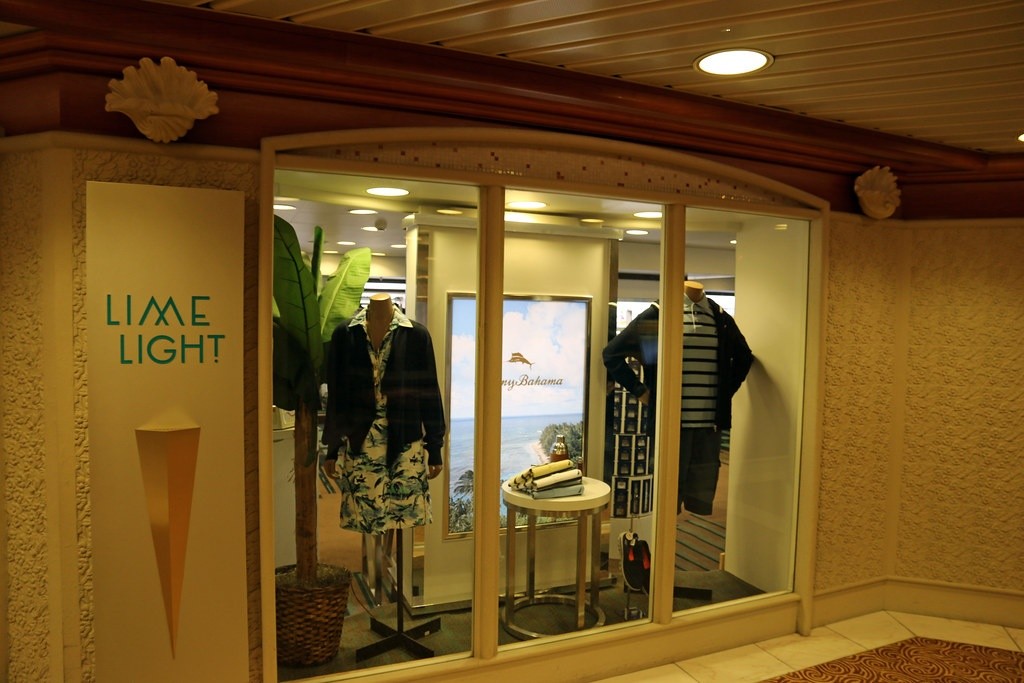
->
<box><xmin>602</xmin><ymin>280</ymin><xmax>753</xmax><ymax>515</ymax></box>
<box><xmin>324</xmin><ymin>295</ymin><xmax>445</xmax><ymax>531</ymax></box>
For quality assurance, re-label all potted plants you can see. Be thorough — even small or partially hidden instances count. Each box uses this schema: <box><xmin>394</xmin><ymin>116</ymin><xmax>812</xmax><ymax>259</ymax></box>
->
<box><xmin>271</xmin><ymin>211</ymin><xmax>372</xmax><ymax>670</ymax></box>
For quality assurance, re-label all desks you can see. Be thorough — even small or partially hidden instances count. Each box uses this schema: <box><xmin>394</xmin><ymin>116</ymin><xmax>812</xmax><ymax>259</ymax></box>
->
<box><xmin>502</xmin><ymin>473</ymin><xmax>611</xmax><ymax>642</ymax></box>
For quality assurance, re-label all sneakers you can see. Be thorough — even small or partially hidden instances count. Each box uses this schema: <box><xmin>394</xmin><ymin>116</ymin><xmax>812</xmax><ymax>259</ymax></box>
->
<box><xmin>617</xmin><ymin>532</ymin><xmax>650</xmax><ymax>594</ymax></box>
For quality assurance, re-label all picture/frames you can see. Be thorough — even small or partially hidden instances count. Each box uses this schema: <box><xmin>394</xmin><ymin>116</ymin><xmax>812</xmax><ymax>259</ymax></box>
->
<box><xmin>321</xmin><ymin>275</ymin><xmax>406</xmax><ymax>313</ymax></box>
